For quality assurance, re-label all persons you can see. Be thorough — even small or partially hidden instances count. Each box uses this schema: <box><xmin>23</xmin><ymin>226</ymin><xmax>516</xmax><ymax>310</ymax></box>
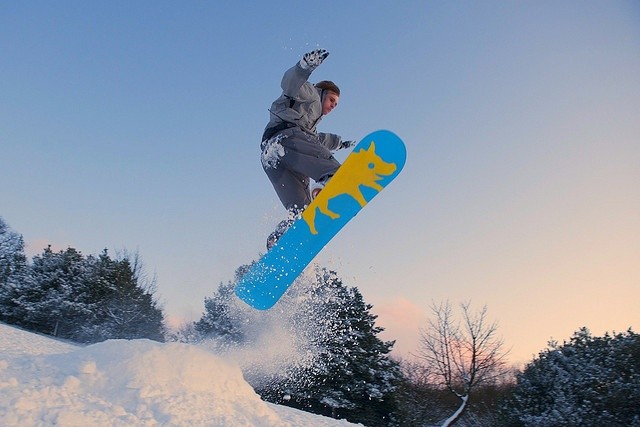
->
<box><xmin>259</xmin><ymin>48</ymin><xmax>358</xmax><ymax>253</ymax></box>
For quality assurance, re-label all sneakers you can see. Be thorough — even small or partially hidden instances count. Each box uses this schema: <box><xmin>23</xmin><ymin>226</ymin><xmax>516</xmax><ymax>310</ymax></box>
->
<box><xmin>310</xmin><ymin>180</ymin><xmax>327</xmax><ymax>201</ymax></box>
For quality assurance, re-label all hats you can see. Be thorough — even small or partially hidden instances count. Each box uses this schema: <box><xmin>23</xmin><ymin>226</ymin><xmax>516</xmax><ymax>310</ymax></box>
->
<box><xmin>316</xmin><ymin>80</ymin><xmax>340</xmax><ymax>96</ymax></box>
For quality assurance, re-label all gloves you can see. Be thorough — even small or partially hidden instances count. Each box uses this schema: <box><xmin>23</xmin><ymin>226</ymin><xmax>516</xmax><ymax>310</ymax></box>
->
<box><xmin>340</xmin><ymin>140</ymin><xmax>356</xmax><ymax>151</ymax></box>
<box><xmin>300</xmin><ymin>49</ymin><xmax>329</xmax><ymax>71</ymax></box>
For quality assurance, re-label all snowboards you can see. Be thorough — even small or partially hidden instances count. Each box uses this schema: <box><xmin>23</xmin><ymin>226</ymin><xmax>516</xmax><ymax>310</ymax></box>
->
<box><xmin>234</xmin><ymin>131</ymin><xmax>407</xmax><ymax>311</ymax></box>
